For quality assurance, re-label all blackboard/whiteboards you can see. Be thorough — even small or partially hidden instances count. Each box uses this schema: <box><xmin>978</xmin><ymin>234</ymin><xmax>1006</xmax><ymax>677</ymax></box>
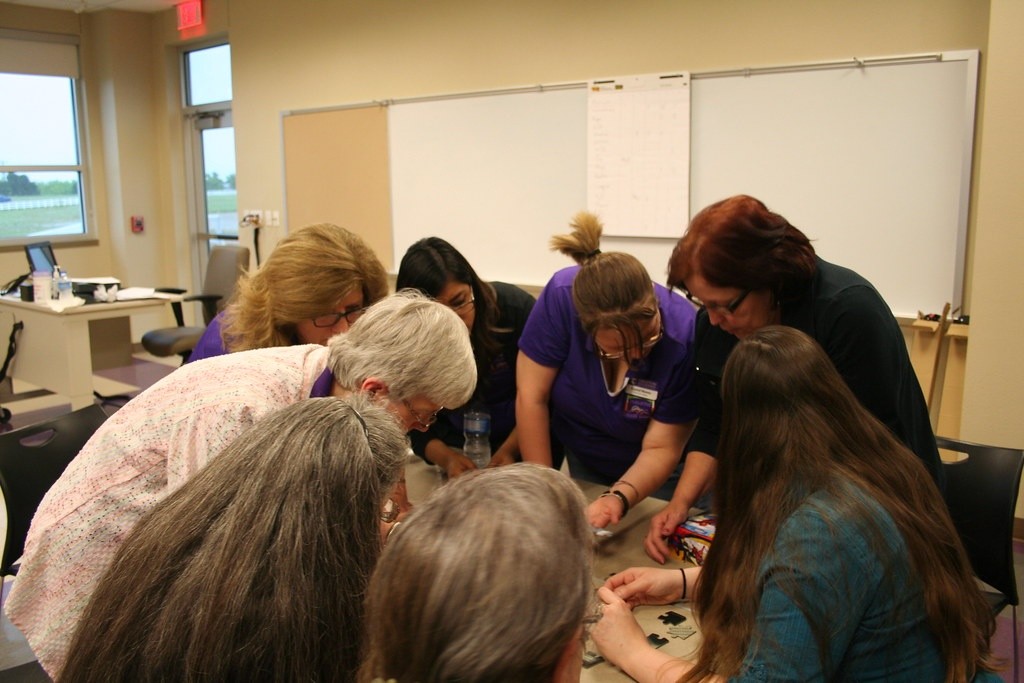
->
<box><xmin>588</xmin><ymin>73</ymin><xmax>691</xmax><ymax>238</ymax></box>
<box><xmin>282</xmin><ymin>48</ymin><xmax>980</xmax><ymax>321</ymax></box>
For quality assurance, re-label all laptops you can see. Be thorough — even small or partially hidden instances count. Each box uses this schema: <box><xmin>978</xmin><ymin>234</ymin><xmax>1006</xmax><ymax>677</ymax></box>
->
<box><xmin>25</xmin><ymin>241</ymin><xmax>60</xmax><ymax>279</ymax></box>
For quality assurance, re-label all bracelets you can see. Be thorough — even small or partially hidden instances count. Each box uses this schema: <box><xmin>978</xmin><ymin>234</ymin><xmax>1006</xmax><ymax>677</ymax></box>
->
<box><xmin>599</xmin><ymin>490</ymin><xmax>629</xmax><ymax>519</ymax></box>
<box><xmin>680</xmin><ymin>568</ymin><xmax>687</xmax><ymax>601</ymax></box>
<box><xmin>613</xmin><ymin>481</ymin><xmax>639</xmax><ymax>503</ymax></box>
<box><xmin>386</xmin><ymin>521</ymin><xmax>401</xmax><ymax>546</ymax></box>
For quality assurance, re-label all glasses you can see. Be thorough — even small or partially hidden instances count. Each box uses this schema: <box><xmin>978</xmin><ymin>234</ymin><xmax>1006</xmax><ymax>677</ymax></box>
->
<box><xmin>686</xmin><ymin>289</ymin><xmax>754</xmax><ymax>314</ymax></box>
<box><xmin>452</xmin><ymin>284</ymin><xmax>476</xmax><ymax>316</ymax></box>
<box><xmin>312</xmin><ymin>308</ymin><xmax>364</xmax><ymax>328</ymax></box>
<box><xmin>379</xmin><ymin>497</ymin><xmax>400</xmax><ymax>523</ymax></box>
<box><xmin>598</xmin><ymin>320</ymin><xmax>664</xmax><ymax>360</ymax></box>
<box><xmin>399</xmin><ymin>393</ymin><xmax>444</xmax><ymax>429</ymax></box>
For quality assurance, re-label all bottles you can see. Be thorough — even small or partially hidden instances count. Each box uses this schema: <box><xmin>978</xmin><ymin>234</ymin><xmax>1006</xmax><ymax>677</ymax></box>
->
<box><xmin>57</xmin><ymin>272</ymin><xmax>74</xmax><ymax>307</ymax></box>
<box><xmin>462</xmin><ymin>405</ymin><xmax>491</xmax><ymax>470</ymax></box>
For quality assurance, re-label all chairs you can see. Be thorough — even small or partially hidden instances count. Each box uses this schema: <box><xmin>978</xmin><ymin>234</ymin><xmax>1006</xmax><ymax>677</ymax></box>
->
<box><xmin>934</xmin><ymin>433</ymin><xmax>1024</xmax><ymax>683</ymax></box>
<box><xmin>0</xmin><ymin>403</ymin><xmax>108</xmax><ymax>578</ymax></box>
<box><xmin>142</xmin><ymin>245</ymin><xmax>251</xmax><ymax>367</ymax></box>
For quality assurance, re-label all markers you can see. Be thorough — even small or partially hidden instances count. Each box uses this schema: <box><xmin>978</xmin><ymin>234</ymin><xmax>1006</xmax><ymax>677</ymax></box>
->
<box><xmin>591</xmin><ymin>85</ymin><xmax>623</xmax><ymax>90</ymax></box>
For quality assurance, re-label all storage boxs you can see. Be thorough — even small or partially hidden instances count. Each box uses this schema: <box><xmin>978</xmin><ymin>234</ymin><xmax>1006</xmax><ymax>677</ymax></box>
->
<box><xmin>666</xmin><ymin>513</ymin><xmax>717</xmax><ymax>565</ymax></box>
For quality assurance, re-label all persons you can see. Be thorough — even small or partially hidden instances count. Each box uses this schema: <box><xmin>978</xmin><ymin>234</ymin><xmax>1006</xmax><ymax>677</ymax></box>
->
<box><xmin>641</xmin><ymin>196</ymin><xmax>946</xmax><ymax>563</ymax></box>
<box><xmin>395</xmin><ymin>238</ymin><xmax>567</xmax><ymax>479</ymax></box>
<box><xmin>58</xmin><ymin>388</ymin><xmax>406</xmax><ymax>683</ymax></box>
<box><xmin>191</xmin><ymin>224</ymin><xmax>415</xmax><ymax>515</ymax></box>
<box><xmin>514</xmin><ymin>212</ymin><xmax>712</xmax><ymax>513</ymax></box>
<box><xmin>3</xmin><ymin>288</ymin><xmax>479</xmax><ymax>682</ymax></box>
<box><xmin>591</xmin><ymin>327</ymin><xmax>997</xmax><ymax>683</ymax></box>
<box><xmin>352</xmin><ymin>460</ymin><xmax>593</xmax><ymax>683</ymax></box>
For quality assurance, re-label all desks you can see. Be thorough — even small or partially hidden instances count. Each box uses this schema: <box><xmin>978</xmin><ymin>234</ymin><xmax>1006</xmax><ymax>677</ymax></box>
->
<box><xmin>385</xmin><ymin>456</ymin><xmax>702</xmax><ymax>683</ymax></box>
<box><xmin>0</xmin><ymin>292</ymin><xmax>185</xmax><ymax>412</ymax></box>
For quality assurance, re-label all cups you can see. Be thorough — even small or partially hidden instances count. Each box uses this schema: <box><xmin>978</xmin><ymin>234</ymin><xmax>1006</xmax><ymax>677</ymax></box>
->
<box><xmin>20</xmin><ymin>285</ymin><xmax>34</xmax><ymax>302</ymax></box>
<box><xmin>33</xmin><ymin>270</ymin><xmax>53</xmax><ymax>305</ymax></box>
